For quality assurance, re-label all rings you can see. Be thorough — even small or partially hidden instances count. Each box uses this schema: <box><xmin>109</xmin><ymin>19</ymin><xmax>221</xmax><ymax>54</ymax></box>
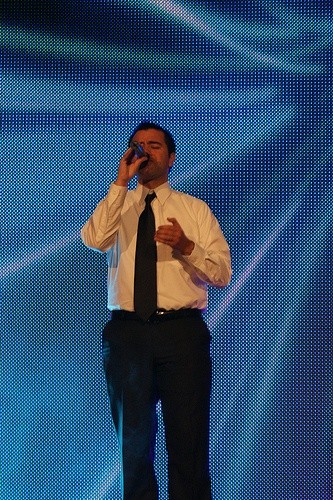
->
<box><xmin>123</xmin><ymin>154</ymin><xmax>127</xmax><ymax>160</ymax></box>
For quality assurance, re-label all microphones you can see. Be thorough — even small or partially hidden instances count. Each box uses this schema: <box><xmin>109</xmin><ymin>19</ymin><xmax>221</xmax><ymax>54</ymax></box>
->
<box><xmin>132</xmin><ymin>142</ymin><xmax>149</xmax><ymax>166</ymax></box>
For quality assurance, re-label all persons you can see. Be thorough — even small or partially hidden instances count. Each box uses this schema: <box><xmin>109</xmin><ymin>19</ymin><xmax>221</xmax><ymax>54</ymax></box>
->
<box><xmin>81</xmin><ymin>120</ymin><xmax>231</xmax><ymax>500</ymax></box>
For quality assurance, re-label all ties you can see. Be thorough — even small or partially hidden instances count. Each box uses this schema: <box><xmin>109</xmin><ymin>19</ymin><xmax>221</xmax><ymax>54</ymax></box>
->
<box><xmin>133</xmin><ymin>191</ymin><xmax>158</xmax><ymax>340</ymax></box>
